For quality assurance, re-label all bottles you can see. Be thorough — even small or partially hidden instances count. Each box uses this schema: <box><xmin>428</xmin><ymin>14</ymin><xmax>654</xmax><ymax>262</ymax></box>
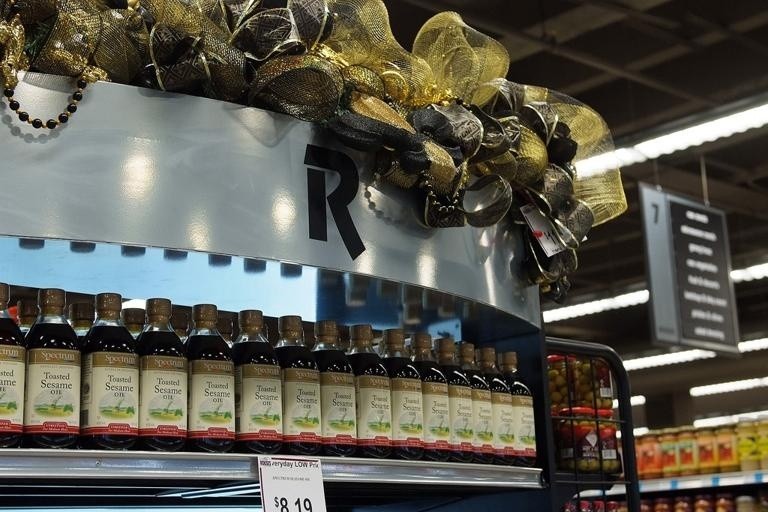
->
<box><xmin>477</xmin><ymin>346</ymin><xmax>513</xmax><ymax>465</ymax></box>
<box><xmin>0</xmin><ymin>283</ymin><xmax>26</xmax><ymax>449</ymax></box>
<box><xmin>136</xmin><ymin>298</ymin><xmax>188</xmax><ymax>452</ymax></box>
<box><xmin>310</xmin><ymin>319</ymin><xmax>357</xmax><ymax>457</ymax></box>
<box><xmin>460</xmin><ymin>342</ymin><xmax>493</xmax><ymax>463</ymax></box>
<box><xmin>433</xmin><ymin>337</ymin><xmax>475</xmax><ymax>463</ymax></box>
<box><xmin>566</xmin><ymin>487</ymin><xmax>768</xmax><ymax>512</ymax></box>
<box><xmin>272</xmin><ymin>315</ymin><xmax>323</xmax><ymax>458</ymax></box>
<box><xmin>231</xmin><ymin>309</ymin><xmax>283</xmax><ymax>455</ymax></box>
<box><xmin>80</xmin><ymin>291</ymin><xmax>140</xmax><ymax>454</ymax></box>
<box><xmin>183</xmin><ymin>303</ymin><xmax>237</xmax><ymax>453</ymax></box>
<box><xmin>496</xmin><ymin>351</ymin><xmax>538</xmax><ymax>467</ymax></box>
<box><xmin>23</xmin><ymin>287</ymin><xmax>83</xmax><ymax>451</ymax></box>
<box><xmin>346</xmin><ymin>323</ymin><xmax>392</xmax><ymax>458</ymax></box>
<box><xmin>408</xmin><ymin>331</ymin><xmax>450</xmax><ymax>464</ymax></box>
<box><xmin>380</xmin><ymin>328</ymin><xmax>423</xmax><ymax>460</ymax></box>
<box><xmin>12</xmin><ymin>304</ymin><xmax>443</xmax><ymax>359</ymax></box>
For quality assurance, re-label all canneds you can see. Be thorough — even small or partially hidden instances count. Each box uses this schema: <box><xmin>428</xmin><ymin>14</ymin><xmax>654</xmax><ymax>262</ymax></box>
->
<box><xmin>547</xmin><ymin>352</ymin><xmax>768</xmax><ymax>512</ymax></box>
